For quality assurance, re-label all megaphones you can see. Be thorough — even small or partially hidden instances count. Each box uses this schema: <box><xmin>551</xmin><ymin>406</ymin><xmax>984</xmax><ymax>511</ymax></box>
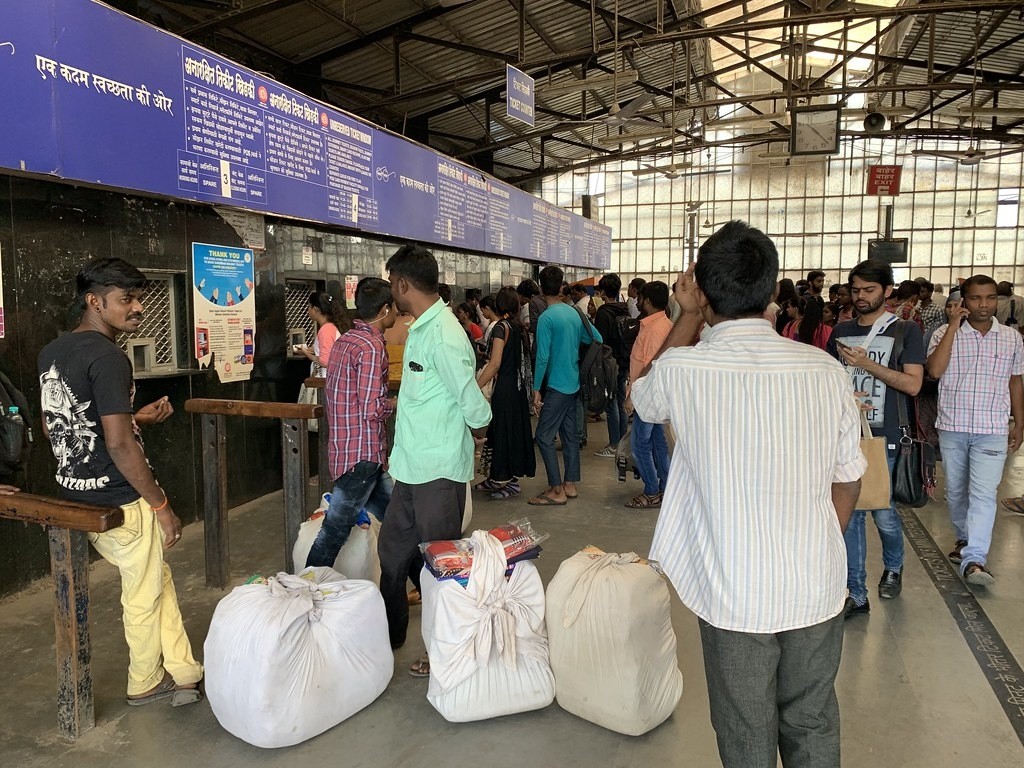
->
<box><xmin>864</xmin><ymin>112</ymin><xmax>885</xmax><ymax>133</ymax></box>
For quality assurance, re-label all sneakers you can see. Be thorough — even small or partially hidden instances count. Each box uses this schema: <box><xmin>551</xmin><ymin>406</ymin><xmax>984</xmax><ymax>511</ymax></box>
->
<box><xmin>594</xmin><ymin>446</ymin><xmax>616</xmax><ymax>457</ymax></box>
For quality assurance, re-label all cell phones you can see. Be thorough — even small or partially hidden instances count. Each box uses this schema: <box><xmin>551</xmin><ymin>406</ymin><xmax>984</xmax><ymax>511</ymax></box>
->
<box><xmin>959</xmin><ymin>301</ymin><xmax>966</xmax><ymax>327</ymax></box>
<box><xmin>835</xmin><ymin>339</ymin><xmax>852</xmax><ymax>351</ymax></box>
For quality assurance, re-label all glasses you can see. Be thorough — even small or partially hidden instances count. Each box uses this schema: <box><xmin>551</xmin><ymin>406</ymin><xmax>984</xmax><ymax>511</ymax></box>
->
<box><xmin>836</xmin><ymin>293</ymin><xmax>850</xmax><ymax>298</ymax></box>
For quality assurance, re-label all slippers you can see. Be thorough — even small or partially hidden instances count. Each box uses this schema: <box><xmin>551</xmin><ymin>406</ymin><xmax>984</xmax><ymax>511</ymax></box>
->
<box><xmin>528</xmin><ymin>494</ymin><xmax>567</xmax><ymax>505</ymax></box>
<box><xmin>170</xmin><ymin>684</ymin><xmax>202</xmax><ymax>707</ymax></box>
<box><xmin>1001</xmin><ymin>496</ymin><xmax>1024</xmax><ymax>515</ymax></box>
<box><xmin>491</xmin><ymin>487</ymin><xmax>521</xmax><ymax>499</ymax></box>
<box><xmin>473</xmin><ymin>477</ymin><xmax>502</xmax><ymax>490</ymax></box>
<box><xmin>568</xmin><ymin>495</ymin><xmax>577</xmax><ymax>499</ymax></box>
<box><xmin>127</xmin><ymin>676</ymin><xmax>176</xmax><ymax>705</ymax></box>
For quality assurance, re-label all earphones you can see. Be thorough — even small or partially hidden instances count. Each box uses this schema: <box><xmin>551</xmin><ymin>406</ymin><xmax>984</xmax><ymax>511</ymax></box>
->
<box><xmin>386</xmin><ymin>309</ymin><xmax>388</xmax><ymax>313</ymax></box>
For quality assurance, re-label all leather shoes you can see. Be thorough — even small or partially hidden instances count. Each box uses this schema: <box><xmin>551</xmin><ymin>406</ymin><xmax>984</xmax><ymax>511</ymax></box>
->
<box><xmin>878</xmin><ymin>565</ymin><xmax>904</xmax><ymax>598</ymax></box>
<box><xmin>844</xmin><ymin>598</ymin><xmax>870</xmax><ymax>620</ymax></box>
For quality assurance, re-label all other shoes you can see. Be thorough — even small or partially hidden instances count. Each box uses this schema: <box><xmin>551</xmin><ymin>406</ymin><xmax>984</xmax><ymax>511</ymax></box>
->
<box><xmin>407</xmin><ymin>587</ymin><xmax>422</xmax><ymax>604</ymax></box>
<box><xmin>533</xmin><ymin>435</ymin><xmax>558</xmax><ymax>445</ymax></box>
<box><xmin>586</xmin><ymin>413</ymin><xmax>603</xmax><ymax>422</ymax></box>
<box><xmin>555</xmin><ymin>438</ymin><xmax>587</xmax><ymax>450</ymax></box>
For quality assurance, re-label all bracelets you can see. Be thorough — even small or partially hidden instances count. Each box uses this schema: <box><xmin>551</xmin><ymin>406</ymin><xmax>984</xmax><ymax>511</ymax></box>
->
<box><xmin>151</xmin><ymin>497</ymin><xmax>168</xmax><ymax>510</ymax></box>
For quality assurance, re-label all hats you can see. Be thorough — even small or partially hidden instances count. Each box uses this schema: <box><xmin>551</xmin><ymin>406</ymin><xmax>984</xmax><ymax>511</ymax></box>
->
<box><xmin>946</xmin><ymin>291</ymin><xmax>960</xmax><ymax>302</ymax></box>
<box><xmin>592</xmin><ymin>297</ymin><xmax>605</xmax><ymax>311</ymax></box>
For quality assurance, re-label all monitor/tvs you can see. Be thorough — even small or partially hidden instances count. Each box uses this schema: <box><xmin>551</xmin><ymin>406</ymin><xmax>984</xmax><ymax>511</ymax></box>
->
<box><xmin>868</xmin><ymin>238</ymin><xmax>908</xmax><ymax>263</ymax></box>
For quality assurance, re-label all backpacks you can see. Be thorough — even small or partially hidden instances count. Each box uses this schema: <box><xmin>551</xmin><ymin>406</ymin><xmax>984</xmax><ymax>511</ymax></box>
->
<box><xmin>0</xmin><ymin>372</ymin><xmax>34</xmax><ymax>478</ymax></box>
<box><xmin>605</xmin><ymin>307</ymin><xmax>639</xmax><ymax>367</ymax></box>
<box><xmin>573</xmin><ymin>305</ymin><xmax>618</xmax><ymax>413</ymax></box>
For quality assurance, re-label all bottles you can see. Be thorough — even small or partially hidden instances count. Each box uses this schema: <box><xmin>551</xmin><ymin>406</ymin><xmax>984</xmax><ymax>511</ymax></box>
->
<box><xmin>3</xmin><ymin>406</ymin><xmax>23</xmax><ymax>462</ymax></box>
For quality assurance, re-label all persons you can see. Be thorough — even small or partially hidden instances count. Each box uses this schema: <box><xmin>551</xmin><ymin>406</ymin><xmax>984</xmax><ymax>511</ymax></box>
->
<box><xmin>36</xmin><ymin>258</ymin><xmax>203</xmax><ymax>706</ymax></box>
<box><xmin>378</xmin><ymin>245</ymin><xmax>491</xmax><ymax>677</ymax></box>
<box><xmin>439</xmin><ymin>266</ymin><xmax>681</xmax><ymax>508</ymax></box>
<box><xmin>296</xmin><ymin>292</ymin><xmax>351</xmax><ymax>487</ymax></box>
<box><xmin>629</xmin><ymin>221</ymin><xmax>867</xmax><ymax>767</ymax></box>
<box><xmin>768</xmin><ymin>259</ymin><xmax>1024</xmax><ymax>618</ymax></box>
<box><xmin>306</xmin><ymin>276</ymin><xmax>427</xmax><ymax>601</ymax></box>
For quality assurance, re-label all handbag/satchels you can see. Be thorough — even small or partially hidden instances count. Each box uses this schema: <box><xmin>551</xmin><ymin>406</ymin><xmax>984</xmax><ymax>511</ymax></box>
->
<box><xmin>297</xmin><ymin>383</ymin><xmax>318</xmax><ymax>432</ymax></box>
<box><xmin>615</xmin><ymin>430</ymin><xmax>640</xmax><ymax>483</ymax></box>
<box><xmin>476</xmin><ymin>364</ymin><xmax>498</xmax><ymax>398</ymax></box>
<box><xmin>894</xmin><ymin>435</ymin><xmax>937</xmax><ymax>507</ymax></box>
<box><xmin>854</xmin><ymin>397</ymin><xmax>891</xmax><ymax>510</ymax></box>
<box><xmin>1004</xmin><ymin>299</ymin><xmax>1018</xmax><ymax>331</ymax></box>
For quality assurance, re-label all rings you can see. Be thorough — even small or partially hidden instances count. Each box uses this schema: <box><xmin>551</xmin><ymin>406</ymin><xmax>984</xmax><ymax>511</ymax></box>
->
<box><xmin>175</xmin><ymin>534</ymin><xmax>180</xmax><ymax>538</ymax></box>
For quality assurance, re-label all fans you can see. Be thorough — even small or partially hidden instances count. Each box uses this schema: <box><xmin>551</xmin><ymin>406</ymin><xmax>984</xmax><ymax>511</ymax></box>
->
<box><xmin>910</xmin><ymin>21</ymin><xmax>1024</xmax><ymax>167</ymax></box>
<box><xmin>642</xmin><ymin>44</ymin><xmax>736</xmax><ymax>228</ymax></box>
<box><xmin>932</xmin><ymin>134</ymin><xmax>991</xmax><ymax>221</ymax></box>
<box><xmin>529</xmin><ymin>0</ymin><xmax>669</xmax><ymax>125</ymax></box>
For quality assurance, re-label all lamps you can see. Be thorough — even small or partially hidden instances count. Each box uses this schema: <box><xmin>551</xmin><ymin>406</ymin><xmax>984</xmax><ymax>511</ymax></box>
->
<box><xmin>841</xmin><ymin>89</ymin><xmax>918</xmax><ymax>118</ymax></box>
<box><xmin>708</xmin><ymin>111</ymin><xmax>784</xmax><ymax>127</ymax></box>
<box><xmin>956</xmin><ymin>92</ymin><xmax>1024</xmax><ymax>119</ymax></box>
<box><xmin>598</xmin><ymin>128</ymin><xmax>673</xmax><ymax>144</ymax></box>
<box><xmin>542</xmin><ymin>69</ymin><xmax>637</xmax><ymax>97</ymax></box>
<box><xmin>631</xmin><ymin>161</ymin><xmax>691</xmax><ymax>171</ymax></box>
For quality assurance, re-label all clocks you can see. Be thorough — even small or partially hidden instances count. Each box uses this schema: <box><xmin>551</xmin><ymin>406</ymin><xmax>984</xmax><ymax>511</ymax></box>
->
<box><xmin>790</xmin><ymin>103</ymin><xmax>840</xmax><ymax>156</ymax></box>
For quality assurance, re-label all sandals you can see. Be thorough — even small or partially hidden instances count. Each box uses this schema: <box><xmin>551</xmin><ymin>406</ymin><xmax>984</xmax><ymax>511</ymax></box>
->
<box><xmin>408</xmin><ymin>650</ymin><xmax>431</xmax><ymax>676</ymax></box>
<box><xmin>625</xmin><ymin>494</ymin><xmax>661</xmax><ymax>508</ymax></box>
<box><xmin>964</xmin><ymin>562</ymin><xmax>995</xmax><ymax>586</ymax></box>
<box><xmin>949</xmin><ymin>540</ymin><xmax>968</xmax><ymax>564</ymax></box>
